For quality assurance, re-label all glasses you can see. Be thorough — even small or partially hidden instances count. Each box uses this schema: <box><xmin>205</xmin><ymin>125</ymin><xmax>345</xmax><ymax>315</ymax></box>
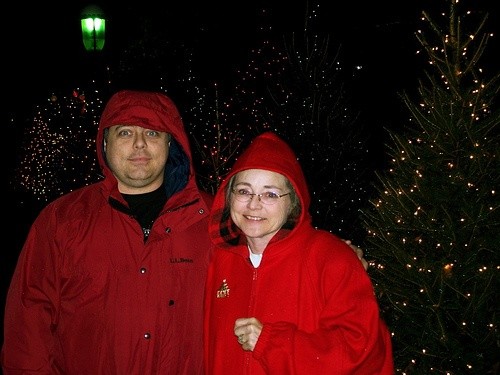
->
<box><xmin>230</xmin><ymin>188</ymin><xmax>293</xmax><ymax>204</ymax></box>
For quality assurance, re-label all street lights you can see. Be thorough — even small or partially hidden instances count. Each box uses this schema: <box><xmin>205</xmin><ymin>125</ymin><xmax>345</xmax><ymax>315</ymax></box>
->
<box><xmin>78</xmin><ymin>0</ymin><xmax>111</xmax><ymax>122</ymax></box>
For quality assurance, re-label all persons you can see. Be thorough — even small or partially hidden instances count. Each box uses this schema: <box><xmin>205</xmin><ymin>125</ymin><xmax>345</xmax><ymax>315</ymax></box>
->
<box><xmin>1</xmin><ymin>88</ymin><xmax>369</xmax><ymax>375</ymax></box>
<box><xmin>205</xmin><ymin>128</ymin><xmax>394</xmax><ymax>375</ymax></box>
